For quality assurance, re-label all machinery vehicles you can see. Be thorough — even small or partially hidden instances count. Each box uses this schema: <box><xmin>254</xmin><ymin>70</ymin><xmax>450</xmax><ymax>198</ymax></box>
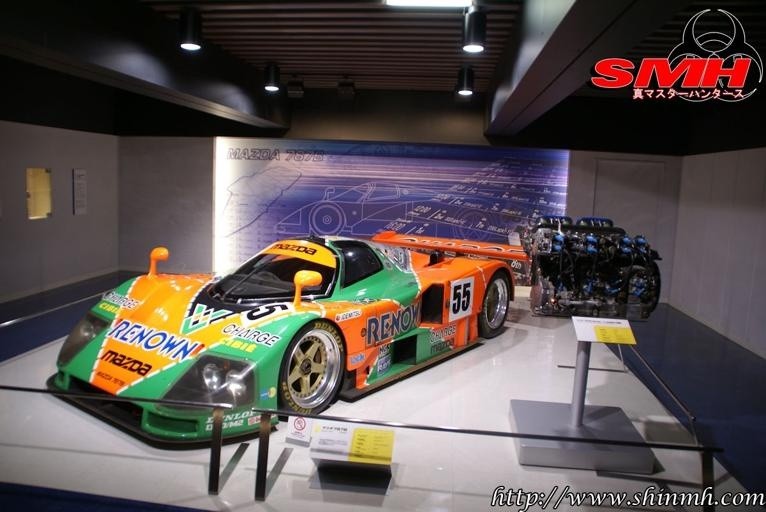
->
<box><xmin>47</xmin><ymin>230</ymin><xmax>534</xmax><ymax>451</ymax></box>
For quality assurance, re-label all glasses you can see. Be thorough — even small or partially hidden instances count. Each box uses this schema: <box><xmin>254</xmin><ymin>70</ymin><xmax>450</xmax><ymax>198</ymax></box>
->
<box><xmin>180</xmin><ymin>10</ymin><xmax>202</xmax><ymax>51</ymax></box>
<box><xmin>462</xmin><ymin>2</ymin><xmax>486</xmax><ymax>53</ymax></box>
<box><xmin>457</xmin><ymin>68</ymin><xmax>474</xmax><ymax>96</ymax></box>
<box><xmin>264</xmin><ymin>64</ymin><xmax>280</xmax><ymax>92</ymax></box>
